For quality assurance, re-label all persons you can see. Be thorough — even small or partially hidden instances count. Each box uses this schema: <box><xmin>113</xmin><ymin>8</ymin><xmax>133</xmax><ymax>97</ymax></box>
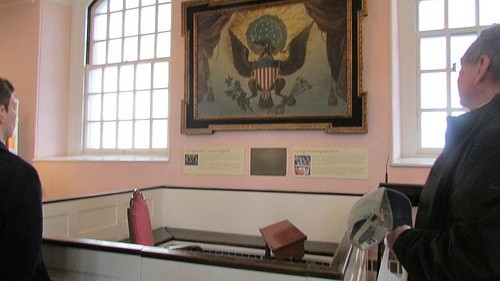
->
<box><xmin>0</xmin><ymin>78</ymin><xmax>50</xmax><ymax>281</ymax></box>
<box><xmin>386</xmin><ymin>22</ymin><xmax>500</xmax><ymax>281</ymax></box>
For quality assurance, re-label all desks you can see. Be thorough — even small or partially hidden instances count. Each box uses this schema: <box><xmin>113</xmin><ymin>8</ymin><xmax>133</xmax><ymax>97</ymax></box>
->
<box><xmin>260</xmin><ymin>218</ymin><xmax>308</xmax><ymax>264</ymax></box>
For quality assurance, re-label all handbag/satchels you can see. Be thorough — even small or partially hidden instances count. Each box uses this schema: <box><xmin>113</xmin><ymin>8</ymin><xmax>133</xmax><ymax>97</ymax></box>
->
<box><xmin>376</xmin><ymin>230</ymin><xmax>409</xmax><ymax>281</ymax></box>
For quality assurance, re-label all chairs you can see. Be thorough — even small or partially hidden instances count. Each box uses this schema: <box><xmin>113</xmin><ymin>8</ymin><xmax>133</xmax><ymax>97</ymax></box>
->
<box><xmin>126</xmin><ymin>186</ymin><xmax>205</xmax><ymax>255</ymax></box>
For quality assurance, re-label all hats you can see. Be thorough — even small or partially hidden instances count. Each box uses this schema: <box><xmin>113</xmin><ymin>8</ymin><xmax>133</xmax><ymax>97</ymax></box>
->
<box><xmin>346</xmin><ymin>186</ymin><xmax>393</xmax><ymax>249</ymax></box>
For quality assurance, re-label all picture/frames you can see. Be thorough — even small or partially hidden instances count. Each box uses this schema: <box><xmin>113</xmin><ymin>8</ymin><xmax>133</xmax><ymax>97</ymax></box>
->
<box><xmin>179</xmin><ymin>0</ymin><xmax>371</xmax><ymax>136</ymax></box>
